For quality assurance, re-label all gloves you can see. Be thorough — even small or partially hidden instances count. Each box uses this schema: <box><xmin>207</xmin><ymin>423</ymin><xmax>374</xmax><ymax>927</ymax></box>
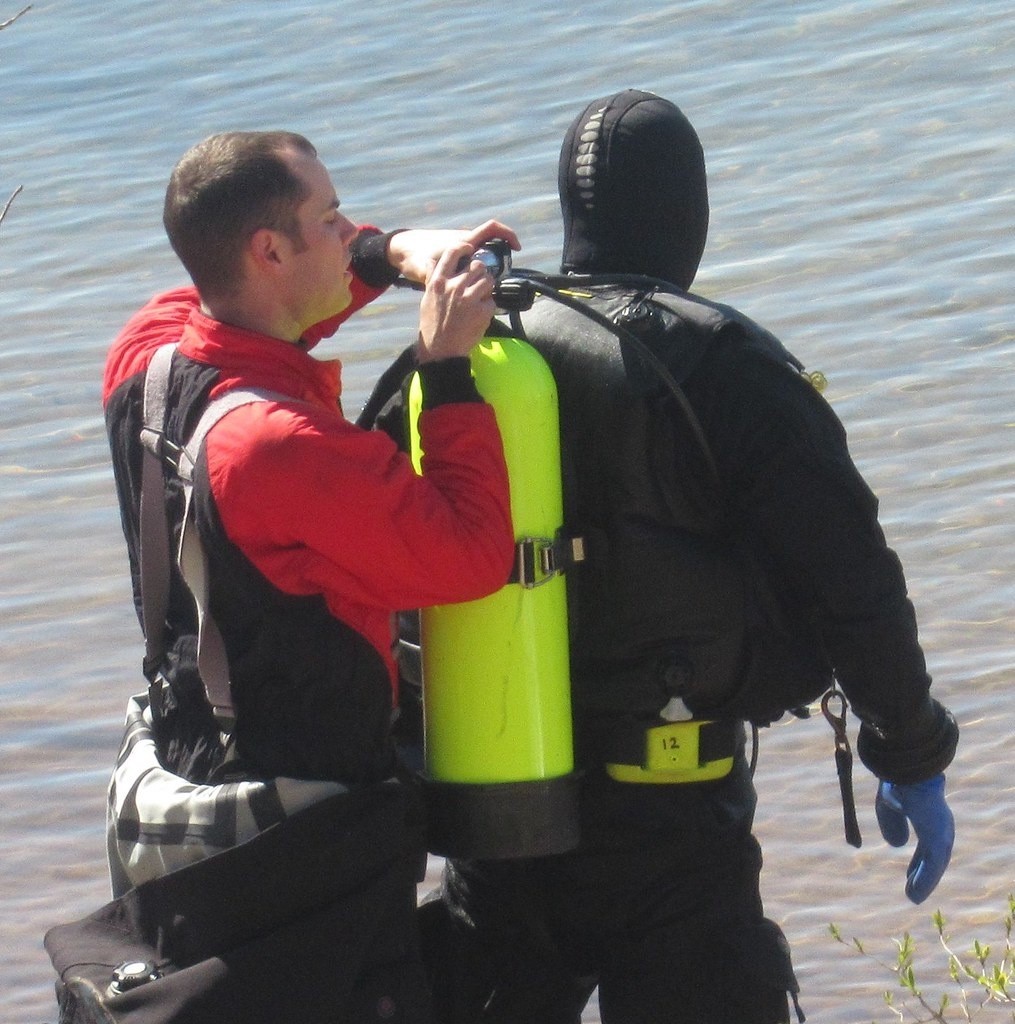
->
<box><xmin>874</xmin><ymin>769</ymin><xmax>955</xmax><ymax>904</ymax></box>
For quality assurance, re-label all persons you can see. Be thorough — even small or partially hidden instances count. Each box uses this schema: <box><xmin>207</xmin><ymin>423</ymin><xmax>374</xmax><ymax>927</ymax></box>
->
<box><xmin>100</xmin><ymin>131</ymin><xmax>522</xmax><ymax>1024</ymax></box>
<box><xmin>363</xmin><ymin>87</ymin><xmax>962</xmax><ymax>1024</ymax></box>
